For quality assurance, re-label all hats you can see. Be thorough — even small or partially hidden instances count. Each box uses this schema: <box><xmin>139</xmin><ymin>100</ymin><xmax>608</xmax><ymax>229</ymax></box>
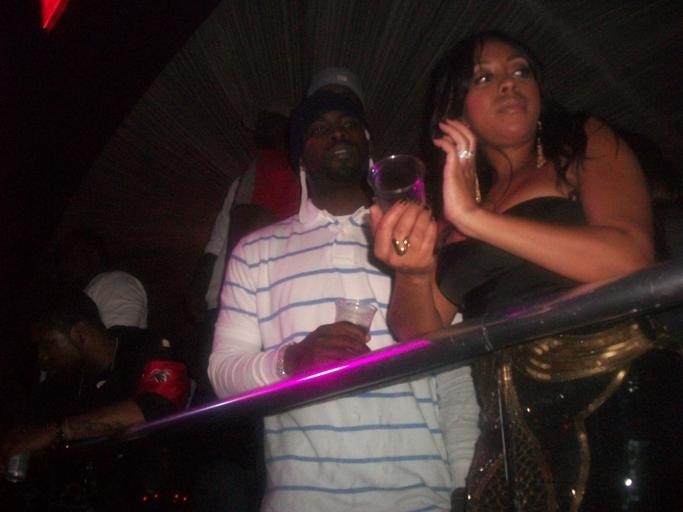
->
<box><xmin>307</xmin><ymin>66</ymin><xmax>366</xmax><ymax>110</ymax></box>
<box><xmin>84</xmin><ymin>270</ymin><xmax>148</xmax><ymax>329</ymax></box>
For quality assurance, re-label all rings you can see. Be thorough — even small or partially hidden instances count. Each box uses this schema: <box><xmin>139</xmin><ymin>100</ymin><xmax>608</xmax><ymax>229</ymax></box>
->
<box><xmin>457</xmin><ymin>149</ymin><xmax>468</xmax><ymax>160</ymax></box>
<box><xmin>467</xmin><ymin>150</ymin><xmax>475</xmax><ymax>159</ymax></box>
<box><xmin>389</xmin><ymin>229</ymin><xmax>409</xmax><ymax>256</ymax></box>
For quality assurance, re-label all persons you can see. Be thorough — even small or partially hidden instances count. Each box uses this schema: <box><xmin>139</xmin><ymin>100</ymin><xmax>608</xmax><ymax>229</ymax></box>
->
<box><xmin>200</xmin><ymin>63</ymin><xmax>484</xmax><ymax>511</ymax></box>
<box><xmin>48</xmin><ymin>221</ymin><xmax>151</xmax><ymax>331</ymax></box>
<box><xmin>195</xmin><ymin>100</ymin><xmax>293</xmax><ymax>381</ymax></box>
<box><xmin>0</xmin><ymin>280</ymin><xmax>191</xmax><ymax>510</ymax></box>
<box><xmin>355</xmin><ymin>21</ymin><xmax>683</xmax><ymax>512</ymax></box>
<box><xmin>206</xmin><ymin>61</ymin><xmax>368</xmax><ymax>324</ymax></box>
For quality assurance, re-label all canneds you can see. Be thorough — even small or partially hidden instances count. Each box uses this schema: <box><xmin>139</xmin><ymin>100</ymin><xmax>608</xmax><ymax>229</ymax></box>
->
<box><xmin>6</xmin><ymin>452</ymin><xmax>30</xmax><ymax>483</ymax></box>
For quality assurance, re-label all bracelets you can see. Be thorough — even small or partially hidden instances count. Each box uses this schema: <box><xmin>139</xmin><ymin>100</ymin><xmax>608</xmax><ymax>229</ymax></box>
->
<box><xmin>55</xmin><ymin>413</ymin><xmax>65</xmax><ymax>448</ymax></box>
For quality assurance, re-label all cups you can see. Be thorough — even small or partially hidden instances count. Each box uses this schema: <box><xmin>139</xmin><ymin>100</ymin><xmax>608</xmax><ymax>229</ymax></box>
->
<box><xmin>333</xmin><ymin>297</ymin><xmax>377</xmax><ymax>335</ymax></box>
<box><xmin>366</xmin><ymin>153</ymin><xmax>429</xmax><ymax>213</ymax></box>
